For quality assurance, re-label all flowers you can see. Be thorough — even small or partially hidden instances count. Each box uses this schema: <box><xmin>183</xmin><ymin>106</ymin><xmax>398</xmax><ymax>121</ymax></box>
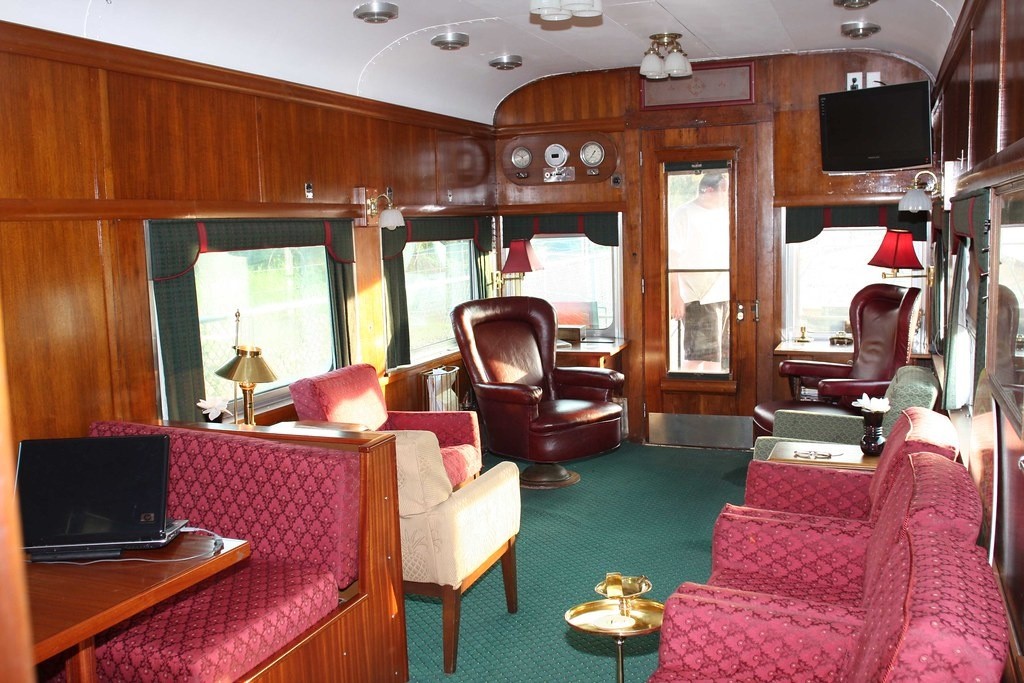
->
<box><xmin>851</xmin><ymin>393</ymin><xmax>890</xmax><ymax>414</ymax></box>
<box><xmin>196</xmin><ymin>393</ymin><xmax>233</xmax><ymax>421</ymax></box>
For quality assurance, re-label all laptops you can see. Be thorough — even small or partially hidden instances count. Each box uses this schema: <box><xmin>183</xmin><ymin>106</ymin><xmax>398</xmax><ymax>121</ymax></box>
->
<box><xmin>13</xmin><ymin>433</ymin><xmax>189</xmax><ymax>553</ymax></box>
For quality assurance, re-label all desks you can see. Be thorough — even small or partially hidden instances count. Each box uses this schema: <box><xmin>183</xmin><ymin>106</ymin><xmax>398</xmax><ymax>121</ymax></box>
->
<box><xmin>25</xmin><ymin>534</ymin><xmax>251</xmax><ymax>683</ymax></box>
<box><xmin>555</xmin><ymin>338</ymin><xmax>628</xmax><ymax>402</ymax></box>
<box><xmin>774</xmin><ymin>341</ymin><xmax>931</xmax><ymax>366</ymax></box>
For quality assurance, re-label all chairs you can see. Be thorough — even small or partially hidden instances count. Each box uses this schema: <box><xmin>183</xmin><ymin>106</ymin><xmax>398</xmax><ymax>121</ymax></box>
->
<box><xmin>651</xmin><ymin>406</ymin><xmax>1008</xmax><ymax>683</ymax></box>
<box><xmin>449</xmin><ymin>296</ymin><xmax>625</xmax><ymax>490</ymax></box>
<box><xmin>752</xmin><ymin>281</ymin><xmax>940</xmax><ymax>460</ymax></box>
<box><xmin>272</xmin><ymin>363</ymin><xmax>522</xmax><ymax>675</ymax></box>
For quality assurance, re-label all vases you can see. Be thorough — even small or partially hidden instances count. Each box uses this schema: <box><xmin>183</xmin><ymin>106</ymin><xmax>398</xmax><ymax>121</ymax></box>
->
<box><xmin>202</xmin><ymin>409</ymin><xmax>223</xmax><ymax>423</ymax></box>
<box><xmin>860</xmin><ymin>411</ymin><xmax>887</xmax><ymax>457</ymax></box>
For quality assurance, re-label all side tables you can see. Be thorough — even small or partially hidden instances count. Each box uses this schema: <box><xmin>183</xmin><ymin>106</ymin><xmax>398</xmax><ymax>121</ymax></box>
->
<box><xmin>767</xmin><ymin>442</ymin><xmax>879</xmax><ymax>471</ymax></box>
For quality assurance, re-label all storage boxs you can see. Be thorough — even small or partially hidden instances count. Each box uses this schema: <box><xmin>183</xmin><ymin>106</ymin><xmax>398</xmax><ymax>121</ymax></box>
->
<box><xmin>557</xmin><ymin>325</ymin><xmax>587</xmax><ymax>342</ymax></box>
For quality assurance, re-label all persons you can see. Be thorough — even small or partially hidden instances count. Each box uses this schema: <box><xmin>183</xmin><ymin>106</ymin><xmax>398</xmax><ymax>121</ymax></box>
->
<box><xmin>668</xmin><ymin>173</ymin><xmax>729</xmax><ymax>370</ymax></box>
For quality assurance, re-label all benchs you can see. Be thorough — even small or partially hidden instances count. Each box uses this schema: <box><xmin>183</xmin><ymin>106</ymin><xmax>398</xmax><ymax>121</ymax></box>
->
<box><xmin>36</xmin><ymin>419</ymin><xmax>410</xmax><ymax>683</ymax></box>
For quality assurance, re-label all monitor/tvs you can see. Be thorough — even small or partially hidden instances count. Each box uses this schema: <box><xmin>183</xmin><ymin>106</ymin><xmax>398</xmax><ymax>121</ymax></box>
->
<box><xmin>818</xmin><ymin>79</ymin><xmax>933</xmax><ymax>174</ymax></box>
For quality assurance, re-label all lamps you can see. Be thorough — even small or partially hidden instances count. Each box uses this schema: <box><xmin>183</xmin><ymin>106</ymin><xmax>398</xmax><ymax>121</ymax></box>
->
<box><xmin>899</xmin><ymin>171</ymin><xmax>942</xmax><ymax>214</ymax></box>
<box><xmin>841</xmin><ymin>23</ymin><xmax>881</xmax><ymax>39</ymax></box>
<box><xmin>214</xmin><ymin>345</ymin><xmax>278</xmax><ymax>426</ymax></box>
<box><xmin>867</xmin><ymin>229</ymin><xmax>935</xmax><ymax>288</ymax></box>
<box><xmin>353</xmin><ymin>2</ymin><xmax>523</xmax><ymax>70</ymax></box>
<box><xmin>529</xmin><ymin>0</ymin><xmax>603</xmax><ymax>22</ymax></box>
<box><xmin>359</xmin><ymin>186</ymin><xmax>406</xmax><ymax>230</ymax></box>
<box><xmin>492</xmin><ymin>240</ymin><xmax>544</xmax><ymax>290</ymax></box>
<box><xmin>639</xmin><ymin>34</ymin><xmax>692</xmax><ymax>79</ymax></box>
